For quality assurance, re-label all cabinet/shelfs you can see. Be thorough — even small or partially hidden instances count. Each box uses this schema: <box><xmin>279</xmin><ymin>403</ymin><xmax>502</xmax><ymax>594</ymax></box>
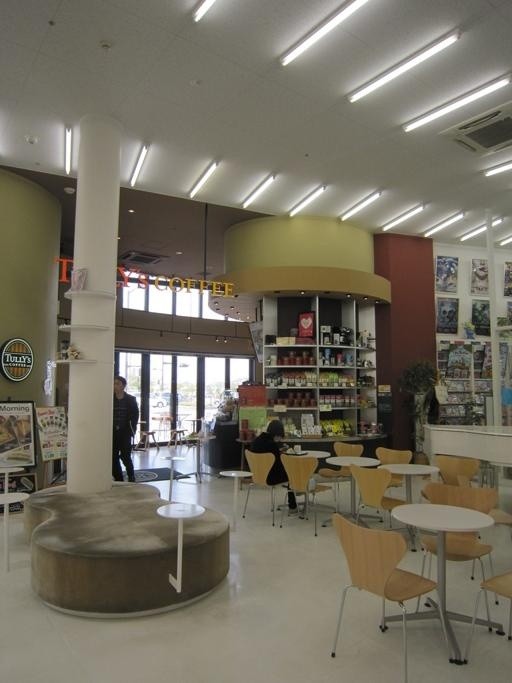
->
<box><xmin>265</xmin><ymin>295</ymin><xmax>377</xmax><ymax>444</ymax></box>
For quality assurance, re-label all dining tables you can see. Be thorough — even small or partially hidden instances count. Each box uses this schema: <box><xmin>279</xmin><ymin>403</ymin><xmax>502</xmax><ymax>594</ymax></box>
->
<box><xmin>396</xmin><ymin>358</ymin><xmax>446</xmax><ymax>465</ymax></box>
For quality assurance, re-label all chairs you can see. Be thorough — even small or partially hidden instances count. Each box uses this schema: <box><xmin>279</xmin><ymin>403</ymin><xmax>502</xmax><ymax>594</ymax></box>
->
<box><xmin>243</xmin><ymin>441</ymin><xmax>482</xmax><ymax>550</ymax></box>
<box><xmin>330</xmin><ymin>512</ymin><xmax>454</xmax><ymax>680</ymax></box>
<box><xmin>465</xmin><ymin>571</ymin><xmax>512</xmax><ymax>664</ymax></box>
<box><xmin>416</xmin><ymin>482</ymin><xmax>498</xmax><ymax>612</ymax></box>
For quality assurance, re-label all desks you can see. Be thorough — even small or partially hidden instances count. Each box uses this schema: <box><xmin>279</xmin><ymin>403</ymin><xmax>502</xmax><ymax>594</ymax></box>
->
<box><xmin>384</xmin><ymin>503</ymin><xmax>505</xmax><ymax>665</ymax></box>
<box><xmin>423</xmin><ymin>425</ymin><xmax>511</xmax><ymax>478</ymax></box>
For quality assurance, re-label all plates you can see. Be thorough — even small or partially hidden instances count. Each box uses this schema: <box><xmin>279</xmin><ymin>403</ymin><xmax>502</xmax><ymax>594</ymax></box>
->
<box><xmin>265</xmin><ymin>295</ymin><xmax>377</xmax><ymax>444</ymax></box>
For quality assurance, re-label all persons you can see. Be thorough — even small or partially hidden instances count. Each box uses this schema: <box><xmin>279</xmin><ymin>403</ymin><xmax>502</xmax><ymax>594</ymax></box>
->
<box><xmin>113</xmin><ymin>375</ymin><xmax>139</xmax><ymax>481</ymax></box>
<box><xmin>249</xmin><ymin>420</ymin><xmax>304</xmax><ymax>512</ymax></box>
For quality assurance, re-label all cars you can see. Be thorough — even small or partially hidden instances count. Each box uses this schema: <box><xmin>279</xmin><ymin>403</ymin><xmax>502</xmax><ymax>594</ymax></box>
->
<box><xmin>294</xmin><ymin>445</ymin><xmax>301</xmax><ymax>453</ymax></box>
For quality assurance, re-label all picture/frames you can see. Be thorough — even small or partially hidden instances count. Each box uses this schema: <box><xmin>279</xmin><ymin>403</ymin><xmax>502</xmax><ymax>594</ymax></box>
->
<box><xmin>1</xmin><ymin>400</ymin><xmax>38</xmax><ymax>468</ymax></box>
<box><xmin>0</xmin><ymin>473</ymin><xmax>38</xmax><ymax>516</ymax></box>
<box><xmin>35</xmin><ymin>406</ymin><xmax>67</xmax><ymax>463</ymax></box>
<box><xmin>423</xmin><ymin>425</ymin><xmax>511</xmax><ymax>478</ymax></box>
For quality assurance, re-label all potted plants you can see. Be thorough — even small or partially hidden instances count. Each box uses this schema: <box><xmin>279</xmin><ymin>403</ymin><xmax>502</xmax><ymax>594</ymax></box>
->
<box><xmin>1</xmin><ymin>400</ymin><xmax>38</xmax><ymax>468</ymax></box>
<box><xmin>0</xmin><ymin>473</ymin><xmax>38</xmax><ymax>516</ymax></box>
<box><xmin>35</xmin><ymin>406</ymin><xmax>67</xmax><ymax>463</ymax></box>
<box><xmin>396</xmin><ymin>358</ymin><xmax>446</xmax><ymax>465</ymax></box>
